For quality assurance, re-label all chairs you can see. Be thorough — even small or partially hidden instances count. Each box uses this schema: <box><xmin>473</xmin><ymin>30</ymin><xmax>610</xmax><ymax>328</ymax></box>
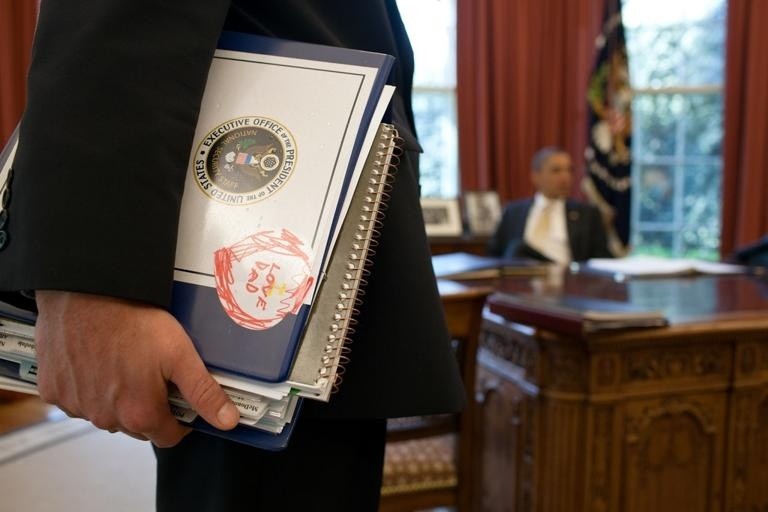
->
<box><xmin>373</xmin><ymin>278</ymin><xmax>499</xmax><ymax>511</ymax></box>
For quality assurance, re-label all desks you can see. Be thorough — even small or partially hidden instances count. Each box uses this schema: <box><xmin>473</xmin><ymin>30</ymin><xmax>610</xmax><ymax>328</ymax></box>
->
<box><xmin>430</xmin><ymin>253</ymin><xmax>768</xmax><ymax>512</ymax></box>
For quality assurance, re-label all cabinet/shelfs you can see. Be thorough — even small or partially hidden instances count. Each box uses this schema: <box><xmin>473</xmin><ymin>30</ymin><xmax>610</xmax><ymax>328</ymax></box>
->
<box><xmin>422</xmin><ymin>234</ymin><xmax>491</xmax><ymax>256</ymax></box>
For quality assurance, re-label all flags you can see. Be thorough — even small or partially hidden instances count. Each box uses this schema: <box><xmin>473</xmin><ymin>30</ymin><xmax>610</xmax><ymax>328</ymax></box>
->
<box><xmin>581</xmin><ymin>0</ymin><xmax>632</xmax><ymax>257</ymax></box>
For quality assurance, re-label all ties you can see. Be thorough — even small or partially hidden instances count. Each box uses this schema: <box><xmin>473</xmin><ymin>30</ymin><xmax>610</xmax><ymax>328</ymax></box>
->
<box><xmin>533</xmin><ymin>200</ymin><xmax>557</xmax><ymax>246</ymax></box>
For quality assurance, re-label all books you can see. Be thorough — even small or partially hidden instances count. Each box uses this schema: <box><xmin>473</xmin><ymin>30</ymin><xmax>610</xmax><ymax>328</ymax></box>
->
<box><xmin>486</xmin><ymin>255</ymin><xmax>546</xmax><ymax>277</ymax></box>
<box><xmin>434</xmin><ymin>251</ymin><xmax>501</xmax><ymax>281</ymax></box>
<box><xmin>487</xmin><ymin>288</ymin><xmax>670</xmax><ymax>344</ymax></box>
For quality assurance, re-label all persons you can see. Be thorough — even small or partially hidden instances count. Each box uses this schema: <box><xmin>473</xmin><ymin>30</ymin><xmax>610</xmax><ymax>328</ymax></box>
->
<box><xmin>13</xmin><ymin>1</ymin><xmax>469</xmax><ymax>511</ymax></box>
<box><xmin>491</xmin><ymin>145</ymin><xmax>616</xmax><ymax>272</ymax></box>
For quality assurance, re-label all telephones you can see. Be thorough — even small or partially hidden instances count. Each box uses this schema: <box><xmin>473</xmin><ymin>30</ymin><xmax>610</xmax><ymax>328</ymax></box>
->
<box><xmin>734</xmin><ymin>236</ymin><xmax>768</xmax><ymax>266</ymax></box>
